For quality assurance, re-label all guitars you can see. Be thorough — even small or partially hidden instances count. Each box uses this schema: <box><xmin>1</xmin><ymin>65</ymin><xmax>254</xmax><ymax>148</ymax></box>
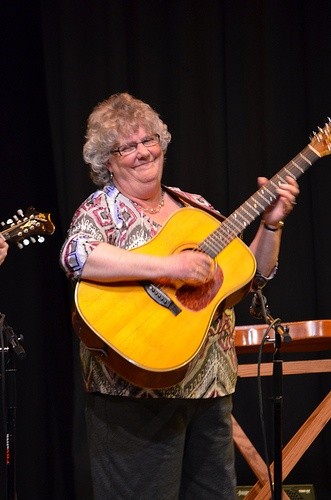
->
<box><xmin>0</xmin><ymin>205</ymin><xmax>56</xmax><ymax>250</ymax></box>
<box><xmin>71</xmin><ymin>117</ymin><xmax>331</xmax><ymax>390</ymax></box>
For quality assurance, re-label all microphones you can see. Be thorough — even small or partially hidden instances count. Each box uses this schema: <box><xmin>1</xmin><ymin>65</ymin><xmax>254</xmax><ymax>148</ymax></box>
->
<box><xmin>249</xmin><ymin>290</ymin><xmax>267</xmax><ymax>320</ymax></box>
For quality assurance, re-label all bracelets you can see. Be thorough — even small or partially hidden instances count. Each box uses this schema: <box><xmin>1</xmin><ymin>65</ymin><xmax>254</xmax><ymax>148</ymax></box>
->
<box><xmin>264</xmin><ymin>223</ymin><xmax>277</xmax><ymax>231</ymax></box>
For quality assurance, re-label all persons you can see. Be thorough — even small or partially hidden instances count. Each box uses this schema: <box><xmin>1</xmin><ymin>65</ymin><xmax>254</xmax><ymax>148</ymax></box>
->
<box><xmin>61</xmin><ymin>92</ymin><xmax>300</xmax><ymax>500</ymax></box>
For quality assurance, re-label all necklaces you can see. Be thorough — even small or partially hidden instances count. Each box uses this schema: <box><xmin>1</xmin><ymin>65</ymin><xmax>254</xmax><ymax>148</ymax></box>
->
<box><xmin>134</xmin><ymin>189</ymin><xmax>164</xmax><ymax>214</ymax></box>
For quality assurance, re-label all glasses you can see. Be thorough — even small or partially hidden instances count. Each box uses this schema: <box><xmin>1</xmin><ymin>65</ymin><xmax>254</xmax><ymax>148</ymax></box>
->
<box><xmin>110</xmin><ymin>132</ymin><xmax>160</xmax><ymax>157</ymax></box>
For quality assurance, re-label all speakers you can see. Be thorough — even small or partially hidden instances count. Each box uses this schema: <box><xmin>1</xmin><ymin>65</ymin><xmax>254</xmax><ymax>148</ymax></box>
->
<box><xmin>237</xmin><ymin>484</ymin><xmax>316</xmax><ymax>500</ymax></box>
